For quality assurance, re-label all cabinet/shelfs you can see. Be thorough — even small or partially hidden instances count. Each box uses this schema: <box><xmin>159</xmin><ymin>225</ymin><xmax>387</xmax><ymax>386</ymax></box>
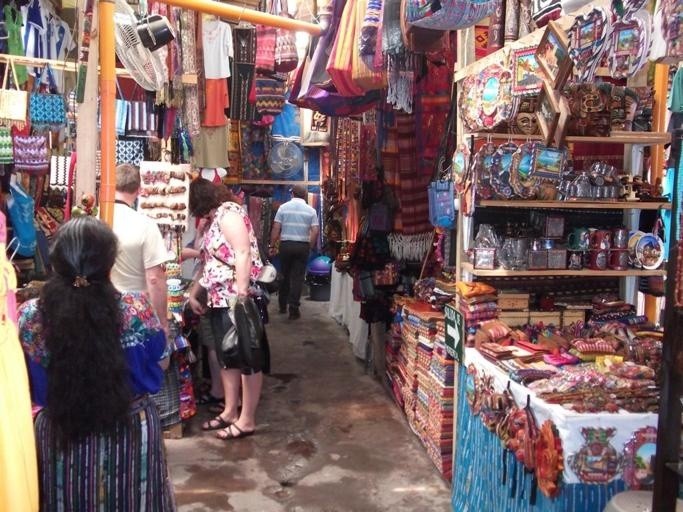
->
<box><xmin>461</xmin><ymin>126</ymin><xmax>674</xmax><ymax>278</ymax></box>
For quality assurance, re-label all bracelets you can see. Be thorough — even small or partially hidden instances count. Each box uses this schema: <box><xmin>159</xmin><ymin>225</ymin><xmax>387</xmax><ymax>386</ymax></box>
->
<box><xmin>268</xmin><ymin>245</ymin><xmax>274</xmax><ymax>248</ymax></box>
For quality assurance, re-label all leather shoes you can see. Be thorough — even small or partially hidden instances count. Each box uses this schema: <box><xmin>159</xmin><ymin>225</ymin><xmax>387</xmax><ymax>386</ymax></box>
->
<box><xmin>280</xmin><ymin>308</ymin><xmax>300</xmax><ymax>319</ymax></box>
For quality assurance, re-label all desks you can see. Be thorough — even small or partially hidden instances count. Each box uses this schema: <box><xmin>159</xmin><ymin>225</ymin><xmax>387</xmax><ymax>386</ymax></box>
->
<box><xmin>449</xmin><ymin>351</ymin><xmax>661</xmax><ymax>512</ymax></box>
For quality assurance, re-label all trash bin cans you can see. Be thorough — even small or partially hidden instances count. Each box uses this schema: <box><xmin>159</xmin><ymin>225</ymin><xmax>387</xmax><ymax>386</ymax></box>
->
<box><xmin>308</xmin><ymin>256</ymin><xmax>331</xmax><ymax>301</ymax></box>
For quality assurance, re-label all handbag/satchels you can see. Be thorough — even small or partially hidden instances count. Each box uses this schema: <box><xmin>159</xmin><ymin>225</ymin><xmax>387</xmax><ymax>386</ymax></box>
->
<box><xmin>254</xmin><ymin>12</ymin><xmax>298</xmax><ymax>114</ymax></box>
<box><xmin>0</xmin><ymin>58</ymin><xmax>158</xmax><ymax>191</ymax></box>
<box><xmin>7</xmin><ymin>182</ymin><xmax>61</xmax><ymax>257</ymax></box>
<box><xmin>256</xmin><ymin>264</ymin><xmax>280</xmax><ymax>293</ymax></box>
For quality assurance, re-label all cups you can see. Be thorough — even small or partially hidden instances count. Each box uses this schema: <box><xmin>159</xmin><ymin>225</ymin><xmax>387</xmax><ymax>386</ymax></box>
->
<box><xmin>567</xmin><ymin>228</ymin><xmax>589</xmax><ymax>250</ymax></box>
<box><xmin>608</xmin><ymin>249</ymin><xmax>629</xmax><ymax>271</ymax></box>
<box><xmin>612</xmin><ymin>228</ymin><xmax>629</xmax><ymax>249</ymax></box>
<box><xmin>583</xmin><ymin>250</ymin><xmax>608</xmax><ymax>271</ymax></box>
<box><xmin>589</xmin><ymin>229</ymin><xmax>612</xmax><ymax>249</ymax></box>
<box><xmin>567</xmin><ymin>250</ymin><xmax>583</xmax><ymax>271</ymax></box>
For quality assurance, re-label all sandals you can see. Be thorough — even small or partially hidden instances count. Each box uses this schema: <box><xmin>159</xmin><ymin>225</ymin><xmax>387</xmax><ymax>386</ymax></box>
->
<box><xmin>193</xmin><ymin>392</ymin><xmax>254</xmax><ymax>438</ymax></box>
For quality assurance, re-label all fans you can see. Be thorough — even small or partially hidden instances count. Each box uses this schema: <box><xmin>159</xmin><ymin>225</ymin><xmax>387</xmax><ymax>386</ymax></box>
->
<box><xmin>111</xmin><ymin>0</ymin><xmax>175</xmax><ymax>94</ymax></box>
<box><xmin>265</xmin><ymin>141</ymin><xmax>303</xmax><ymax>179</ymax></box>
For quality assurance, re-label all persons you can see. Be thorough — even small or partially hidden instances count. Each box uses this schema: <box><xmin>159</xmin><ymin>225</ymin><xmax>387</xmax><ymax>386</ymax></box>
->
<box><xmin>179</xmin><ymin>177</ymin><xmax>270</xmax><ymax>439</ymax></box>
<box><xmin>89</xmin><ymin>164</ymin><xmax>171</xmax><ymax>371</ymax></box>
<box><xmin>268</xmin><ymin>184</ymin><xmax>320</xmax><ymax>320</ymax></box>
<box><xmin>14</xmin><ymin>215</ymin><xmax>175</xmax><ymax>511</ymax></box>
